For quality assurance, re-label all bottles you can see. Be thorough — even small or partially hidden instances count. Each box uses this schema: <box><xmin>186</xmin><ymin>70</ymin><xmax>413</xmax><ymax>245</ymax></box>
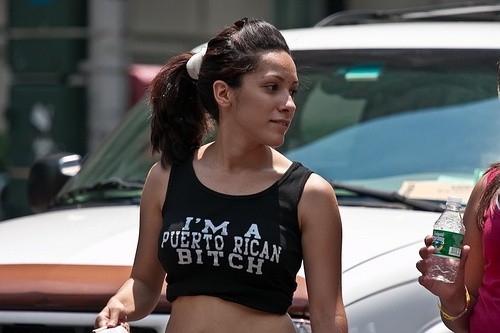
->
<box><xmin>421</xmin><ymin>198</ymin><xmax>469</xmax><ymax>284</ymax></box>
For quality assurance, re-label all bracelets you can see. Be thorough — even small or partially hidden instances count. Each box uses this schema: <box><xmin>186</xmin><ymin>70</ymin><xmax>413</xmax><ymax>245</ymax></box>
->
<box><xmin>437</xmin><ymin>284</ymin><xmax>471</xmax><ymax>321</ymax></box>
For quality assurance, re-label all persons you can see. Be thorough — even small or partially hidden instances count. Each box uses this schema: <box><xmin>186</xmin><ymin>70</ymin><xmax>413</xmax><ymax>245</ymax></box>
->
<box><xmin>417</xmin><ymin>161</ymin><xmax>500</xmax><ymax>333</ymax></box>
<box><xmin>93</xmin><ymin>16</ymin><xmax>348</xmax><ymax>333</ymax></box>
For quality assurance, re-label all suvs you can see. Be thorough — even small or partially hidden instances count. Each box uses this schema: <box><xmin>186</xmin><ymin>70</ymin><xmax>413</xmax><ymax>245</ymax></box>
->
<box><xmin>1</xmin><ymin>3</ymin><xmax>500</xmax><ymax>333</ymax></box>
